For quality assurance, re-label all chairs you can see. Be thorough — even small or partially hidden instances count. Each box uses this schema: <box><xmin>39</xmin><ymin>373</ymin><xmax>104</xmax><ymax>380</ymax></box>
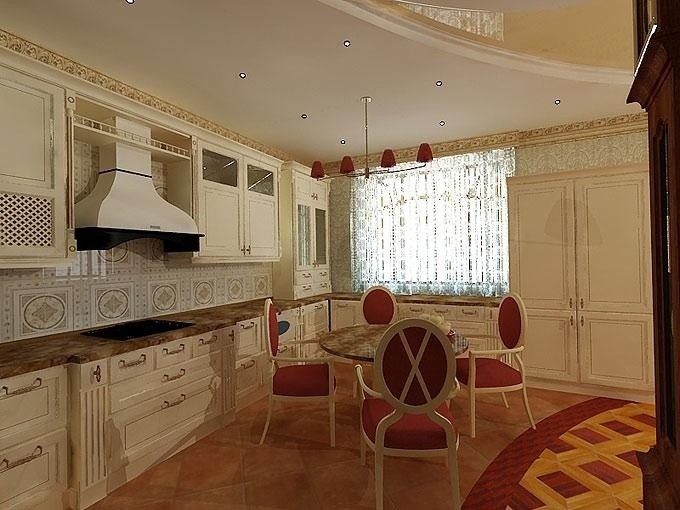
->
<box><xmin>358</xmin><ymin>286</ymin><xmax>400</xmax><ymax>407</ymax></box>
<box><xmin>260</xmin><ymin>300</ymin><xmax>338</xmax><ymax>449</ymax></box>
<box><xmin>354</xmin><ymin>323</ymin><xmax>460</xmax><ymax>508</ymax></box>
<box><xmin>452</xmin><ymin>290</ymin><xmax>536</xmax><ymax>439</ymax></box>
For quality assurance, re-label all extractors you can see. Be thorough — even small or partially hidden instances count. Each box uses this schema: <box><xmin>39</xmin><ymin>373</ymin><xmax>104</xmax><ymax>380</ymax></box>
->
<box><xmin>69</xmin><ymin>118</ymin><xmax>204</xmax><ymax>254</ymax></box>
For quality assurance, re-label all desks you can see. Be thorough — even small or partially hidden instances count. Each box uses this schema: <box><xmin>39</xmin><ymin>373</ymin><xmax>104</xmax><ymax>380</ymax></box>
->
<box><xmin>320</xmin><ymin>321</ymin><xmax>468</xmax><ymax>461</ymax></box>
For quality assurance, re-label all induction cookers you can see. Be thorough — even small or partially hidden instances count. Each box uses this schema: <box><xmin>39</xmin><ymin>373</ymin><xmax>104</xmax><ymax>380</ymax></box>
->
<box><xmin>79</xmin><ymin>318</ymin><xmax>196</xmax><ymax>341</ymax></box>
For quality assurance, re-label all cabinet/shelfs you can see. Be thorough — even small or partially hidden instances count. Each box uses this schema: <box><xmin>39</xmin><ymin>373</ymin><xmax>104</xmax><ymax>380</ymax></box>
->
<box><xmin>506</xmin><ymin>161</ymin><xmax>659</xmax><ymax>406</ymax></box>
<box><xmin>164</xmin><ymin>129</ymin><xmax>284</xmax><ymax>266</ymax></box>
<box><xmin>230</xmin><ymin>306</ymin><xmax>301</xmax><ymax>428</ymax></box>
<box><xmin>68</xmin><ymin>326</ymin><xmax>228</xmax><ymax>510</ymax></box>
<box><xmin>1</xmin><ymin>364</ymin><xmax>76</xmax><ymax>510</ymax></box>
<box><xmin>2</xmin><ymin>46</ymin><xmax>79</xmax><ymax>272</ymax></box>
<box><xmin>272</xmin><ymin>160</ymin><xmax>335</xmax><ymax>301</ymax></box>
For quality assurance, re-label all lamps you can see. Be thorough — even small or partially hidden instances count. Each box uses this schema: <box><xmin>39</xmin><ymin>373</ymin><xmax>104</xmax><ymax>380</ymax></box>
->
<box><xmin>311</xmin><ymin>96</ymin><xmax>433</xmax><ymax>182</ymax></box>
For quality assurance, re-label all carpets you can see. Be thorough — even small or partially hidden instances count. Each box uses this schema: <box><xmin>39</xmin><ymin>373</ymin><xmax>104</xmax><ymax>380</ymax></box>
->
<box><xmin>462</xmin><ymin>393</ymin><xmax>656</xmax><ymax>508</ymax></box>
